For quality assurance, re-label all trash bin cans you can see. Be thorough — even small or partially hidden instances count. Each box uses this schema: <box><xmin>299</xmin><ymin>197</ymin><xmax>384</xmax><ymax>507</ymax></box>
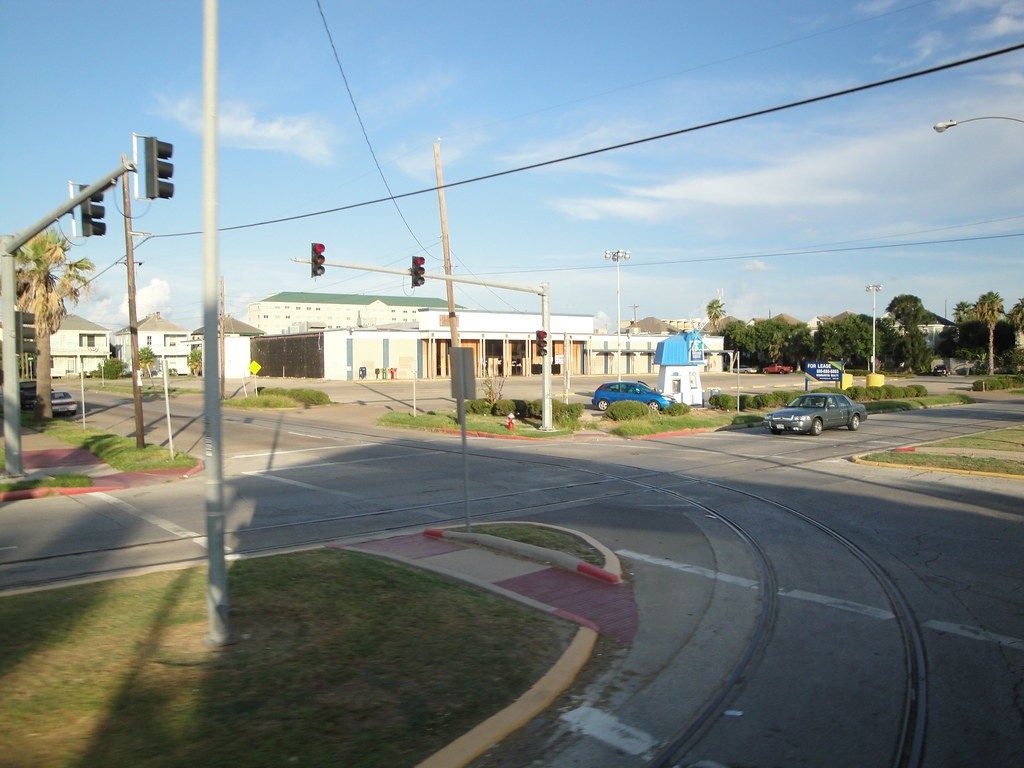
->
<box><xmin>359</xmin><ymin>367</ymin><xmax>366</xmax><ymax>377</ymax></box>
<box><xmin>710</xmin><ymin>387</ymin><xmax>721</xmax><ymax>396</ymax></box>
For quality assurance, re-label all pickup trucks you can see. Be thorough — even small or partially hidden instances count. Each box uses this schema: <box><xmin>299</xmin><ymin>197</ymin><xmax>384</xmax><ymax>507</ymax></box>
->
<box><xmin>763</xmin><ymin>364</ymin><xmax>793</xmax><ymax>374</ymax></box>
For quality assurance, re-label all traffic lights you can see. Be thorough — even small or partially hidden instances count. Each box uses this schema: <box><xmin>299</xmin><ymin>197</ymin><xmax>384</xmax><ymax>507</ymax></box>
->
<box><xmin>80</xmin><ymin>185</ymin><xmax>106</xmax><ymax>237</ymax></box>
<box><xmin>15</xmin><ymin>311</ymin><xmax>36</xmax><ymax>353</ymax></box>
<box><xmin>536</xmin><ymin>331</ymin><xmax>547</xmax><ymax>356</ymax></box>
<box><xmin>412</xmin><ymin>256</ymin><xmax>425</xmax><ymax>288</ymax></box>
<box><xmin>311</xmin><ymin>242</ymin><xmax>325</xmax><ymax>278</ymax></box>
<box><xmin>145</xmin><ymin>137</ymin><xmax>173</xmax><ymax>200</ymax></box>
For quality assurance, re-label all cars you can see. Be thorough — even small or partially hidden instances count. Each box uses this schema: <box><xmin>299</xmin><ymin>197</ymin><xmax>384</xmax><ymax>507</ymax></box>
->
<box><xmin>592</xmin><ymin>382</ymin><xmax>675</xmax><ymax>411</ymax></box>
<box><xmin>733</xmin><ymin>365</ymin><xmax>757</xmax><ymax>374</ymax></box>
<box><xmin>934</xmin><ymin>365</ymin><xmax>951</xmax><ymax>376</ymax></box>
<box><xmin>762</xmin><ymin>393</ymin><xmax>868</xmax><ymax>436</ymax></box>
<box><xmin>49</xmin><ymin>390</ymin><xmax>77</xmax><ymax>415</ymax></box>
<box><xmin>19</xmin><ymin>380</ymin><xmax>38</xmax><ymax>409</ymax></box>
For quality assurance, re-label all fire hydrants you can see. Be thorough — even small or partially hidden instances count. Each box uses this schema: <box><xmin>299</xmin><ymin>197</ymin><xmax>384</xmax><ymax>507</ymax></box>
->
<box><xmin>505</xmin><ymin>413</ymin><xmax>515</xmax><ymax>430</ymax></box>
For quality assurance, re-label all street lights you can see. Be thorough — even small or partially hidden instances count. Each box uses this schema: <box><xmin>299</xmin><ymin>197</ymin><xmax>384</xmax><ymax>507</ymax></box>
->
<box><xmin>866</xmin><ymin>284</ymin><xmax>883</xmax><ymax>374</ymax></box>
<box><xmin>605</xmin><ymin>250</ymin><xmax>629</xmax><ymax>382</ymax></box>
